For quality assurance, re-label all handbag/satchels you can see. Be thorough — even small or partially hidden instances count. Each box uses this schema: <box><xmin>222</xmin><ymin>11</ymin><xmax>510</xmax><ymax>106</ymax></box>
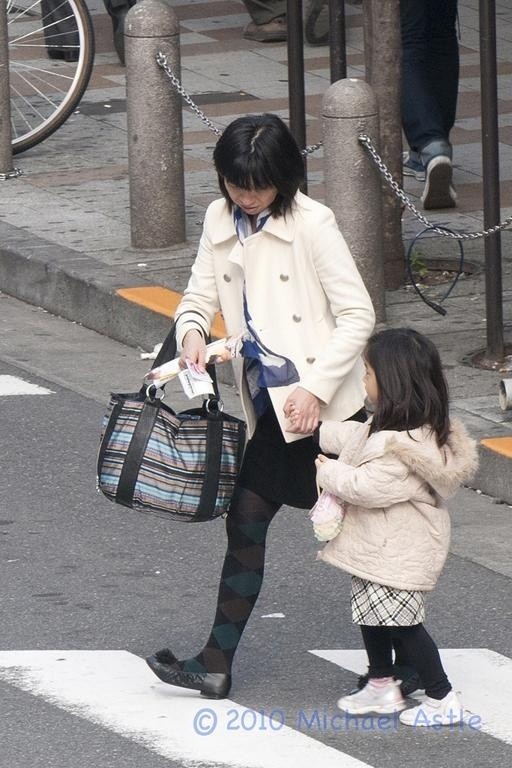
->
<box><xmin>97</xmin><ymin>391</ymin><xmax>246</xmax><ymax>522</ymax></box>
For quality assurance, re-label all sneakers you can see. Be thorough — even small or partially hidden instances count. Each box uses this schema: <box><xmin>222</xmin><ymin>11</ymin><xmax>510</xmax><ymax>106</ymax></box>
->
<box><xmin>337</xmin><ymin>678</ymin><xmax>408</xmax><ymax>715</ymax></box>
<box><xmin>401</xmin><ymin>150</ymin><xmax>458</xmax><ymax>209</ymax></box>
<box><xmin>399</xmin><ymin>691</ymin><xmax>467</xmax><ymax>726</ymax></box>
<box><xmin>244</xmin><ymin>16</ymin><xmax>288</xmax><ymax>43</ymax></box>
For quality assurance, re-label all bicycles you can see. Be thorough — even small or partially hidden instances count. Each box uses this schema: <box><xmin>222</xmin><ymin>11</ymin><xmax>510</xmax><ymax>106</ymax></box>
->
<box><xmin>6</xmin><ymin>1</ymin><xmax>96</xmax><ymax>156</ymax></box>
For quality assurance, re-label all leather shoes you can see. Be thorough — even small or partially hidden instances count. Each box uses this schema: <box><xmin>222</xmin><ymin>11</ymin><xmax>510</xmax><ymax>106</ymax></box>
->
<box><xmin>147</xmin><ymin>649</ymin><xmax>231</xmax><ymax>699</ymax></box>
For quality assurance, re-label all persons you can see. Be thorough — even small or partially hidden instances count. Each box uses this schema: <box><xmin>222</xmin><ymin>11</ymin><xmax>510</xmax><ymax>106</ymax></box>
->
<box><xmin>286</xmin><ymin>326</ymin><xmax>479</xmax><ymax>727</ymax></box>
<box><xmin>145</xmin><ymin>112</ymin><xmax>428</xmax><ymax>700</ymax></box>
<box><xmin>397</xmin><ymin>0</ymin><xmax>460</xmax><ymax>210</ymax></box>
<box><xmin>41</xmin><ymin>1</ymin><xmax>137</xmax><ymax>64</ymax></box>
<box><xmin>240</xmin><ymin>0</ymin><xmax>332</xmax><ymax>46</ymax></box>
